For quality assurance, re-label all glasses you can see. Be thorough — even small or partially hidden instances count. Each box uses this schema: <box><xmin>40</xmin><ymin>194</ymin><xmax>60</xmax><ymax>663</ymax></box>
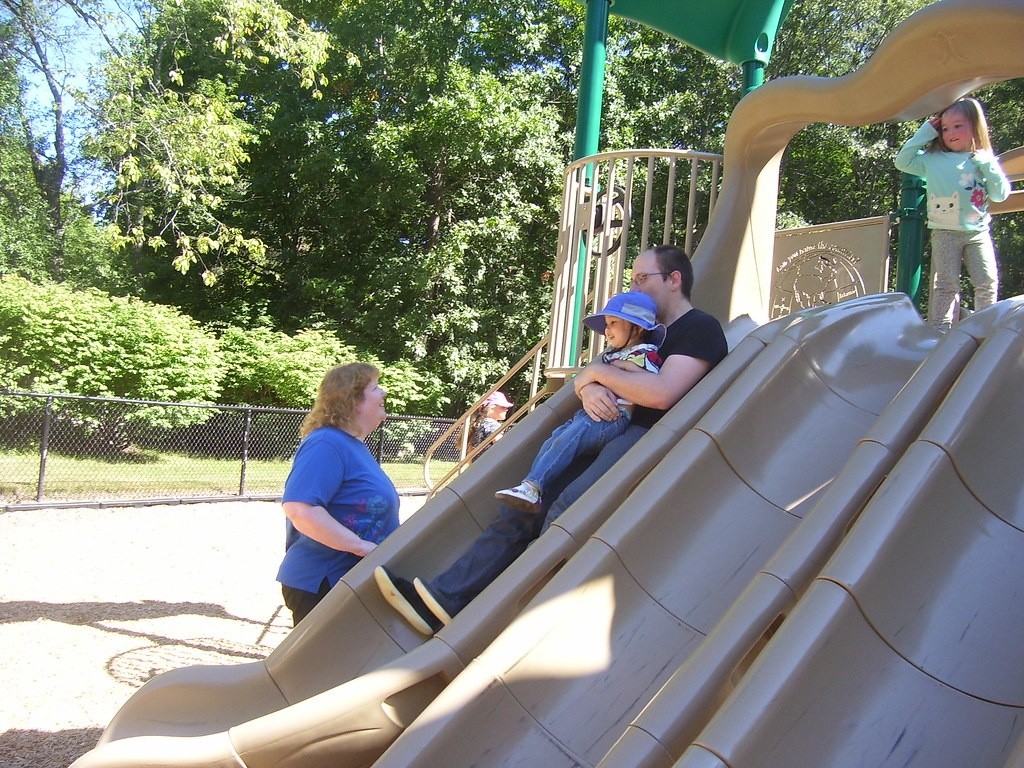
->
<box><xmin>634</xmin><ymin>271</ymin><xmax>672</xmax><ymax>285</ymax></box>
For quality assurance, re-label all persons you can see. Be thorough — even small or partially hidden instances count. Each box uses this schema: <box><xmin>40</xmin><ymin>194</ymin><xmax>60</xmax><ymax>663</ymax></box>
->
<box><xmin>374</xmin><ymin>244</ymin><xmax>730</xmax><ymax>635</ymax></box>
<box><xmin>456</xmin><ymin>391</ymin><xmax>515</xmax><ymax>466</ymax></box>
<box><xmin>494</xmin><ymin>290</ymin><xmax>666</xmax><ymax>515</ymax></box>
<box><xmin>277</xmin><ymin>364</ymin><xmax>400</xmax><ymax>631</ymax></box>
<box><xmin>896</xmin><ymin>99</ymin><xmax>1012</xmax><ymax>337</ymax></box>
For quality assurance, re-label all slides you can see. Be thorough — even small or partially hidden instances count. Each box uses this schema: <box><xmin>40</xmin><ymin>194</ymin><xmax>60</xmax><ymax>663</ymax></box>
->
<box><xmin>668</xmin><ymin>299</ymin><xmax>1024</xmax><ymax>768</ymax></box>
<box><xmin>68</xmin><ymin>312</ymin><xmax>797</xmax><ymax>768</ymax></box>
<box><xmin>370</xmin><ymin>292</ymin><xmax>1024</xmax><ymax>766</ymax></box>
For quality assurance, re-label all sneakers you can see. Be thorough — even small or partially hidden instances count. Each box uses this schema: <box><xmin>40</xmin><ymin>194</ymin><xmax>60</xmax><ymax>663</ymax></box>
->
<box><xmin>374</xmin><ymin>564</ymin><xmax>453</xmax><ymax>636</ymax></box>
<box><xmin>495</xmin><ymin>483</ymin><xmax>543</xmax><ymax>514</ymax></box>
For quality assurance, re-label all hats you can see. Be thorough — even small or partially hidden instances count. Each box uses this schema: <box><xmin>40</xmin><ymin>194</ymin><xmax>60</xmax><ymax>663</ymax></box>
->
<box><xmin>583</xmin><ymin>291</ymin><xmax>668</xmax><ymax>350</ymax></box>
<box><xmin>481</xmin><ymin>391</ymin><xmax>515</xmax><ymax>408</ymax></box>
